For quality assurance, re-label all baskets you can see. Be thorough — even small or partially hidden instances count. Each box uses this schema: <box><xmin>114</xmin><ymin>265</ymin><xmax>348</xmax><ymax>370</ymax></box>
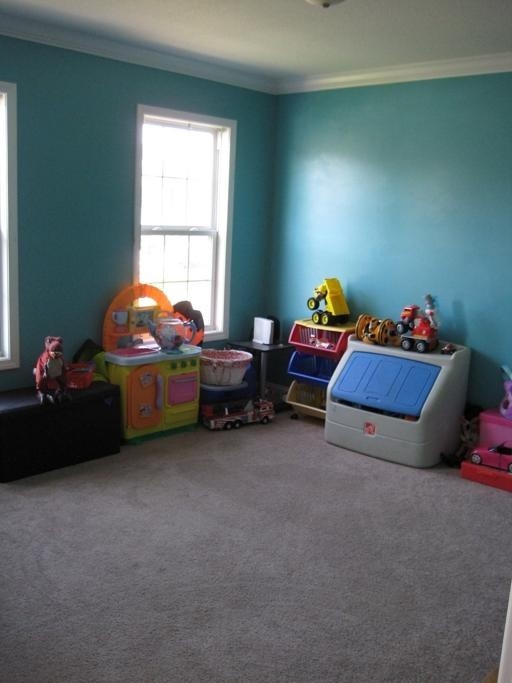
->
<box><xmin>200</xmin><ymin>348</ymin><xmax>253</xmax><ymax>385</ymax></box>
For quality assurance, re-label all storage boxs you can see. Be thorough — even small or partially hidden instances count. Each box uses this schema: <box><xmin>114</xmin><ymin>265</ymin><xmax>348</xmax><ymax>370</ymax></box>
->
<box><xmin>202</xmin><ymin>380</ymin><xmax>247</xmax><ymax>415</ymax></box>
<box><xmin>324</xmin><ymin>334</ymin><xmax>469</xmax><ymax>470</ymax></box>
<box><xmin>477</xmin><ymin>407</ymin><xmax>512</xmax><ymax>449</ymax></box>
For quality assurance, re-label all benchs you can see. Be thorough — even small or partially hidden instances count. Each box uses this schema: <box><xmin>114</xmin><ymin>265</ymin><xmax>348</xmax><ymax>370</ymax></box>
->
<box><xmin>1</xmin><ymin>378</ymin><xmax>122</xmax><ymax>486</ymax></box>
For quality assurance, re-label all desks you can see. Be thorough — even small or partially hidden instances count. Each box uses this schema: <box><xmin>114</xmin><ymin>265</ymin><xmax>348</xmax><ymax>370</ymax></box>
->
<box><xmin>232</xmin><ymin>339</ymin><xmax>295</xmax><ymax>414</ymax></box>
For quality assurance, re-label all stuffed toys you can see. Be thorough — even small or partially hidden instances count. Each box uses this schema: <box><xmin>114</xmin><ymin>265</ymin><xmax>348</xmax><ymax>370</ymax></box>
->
<box><xmin>33</xmin><ymin>336</ymin><xmax>66</xmax><ymax>405</ymax></box>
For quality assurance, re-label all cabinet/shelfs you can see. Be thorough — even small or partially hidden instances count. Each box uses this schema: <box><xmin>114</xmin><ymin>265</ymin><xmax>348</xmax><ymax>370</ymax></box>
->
<box><xmin>285</xmin><ymin>317</ymin><xmax>355</xmax><ymax>419</ymax></box>
